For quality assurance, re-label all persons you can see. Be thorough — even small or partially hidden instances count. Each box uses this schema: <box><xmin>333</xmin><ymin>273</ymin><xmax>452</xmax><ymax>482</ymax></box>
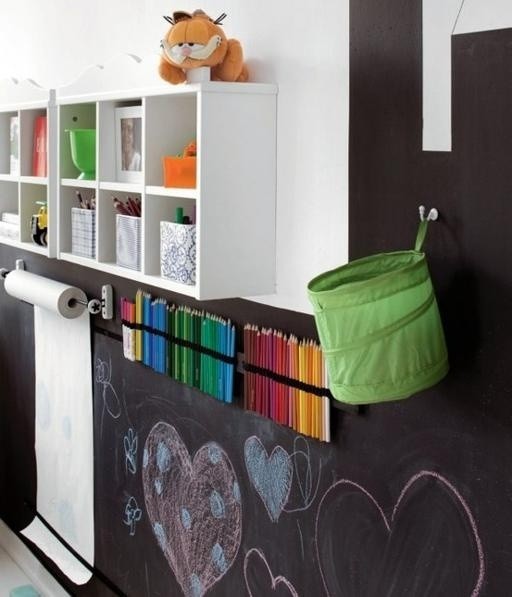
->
<box><xmin>120</xmin><ymin>118</ymin><xmax>142</xmax><ymax>170</ymax></box>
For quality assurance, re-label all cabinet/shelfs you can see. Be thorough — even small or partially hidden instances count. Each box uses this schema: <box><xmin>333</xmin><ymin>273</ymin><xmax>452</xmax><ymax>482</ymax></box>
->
<box><xmin>0</xmin><ymin>77</ymin><xmax>286</xmax><ymax>303</ymax></box>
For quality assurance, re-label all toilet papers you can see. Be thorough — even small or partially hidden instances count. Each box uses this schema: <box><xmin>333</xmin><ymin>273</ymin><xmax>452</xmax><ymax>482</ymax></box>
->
<box><xmin>3</xmin><ymin>269</ymin><xmax>95</xmax><ymax>589</ymax></box>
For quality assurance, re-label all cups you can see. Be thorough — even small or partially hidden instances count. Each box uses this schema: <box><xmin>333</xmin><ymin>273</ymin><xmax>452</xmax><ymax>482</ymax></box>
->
<box><xmin>187</xmin><ymin>65</ymin><xmax>211</xmax><ymax>84</ymax></box>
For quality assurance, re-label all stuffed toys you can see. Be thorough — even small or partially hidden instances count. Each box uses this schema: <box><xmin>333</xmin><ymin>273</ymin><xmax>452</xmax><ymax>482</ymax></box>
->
<box><xmin>158</xmin><ymin>8</ymin><xmax>254</xmax><ymax>85</ymax></box>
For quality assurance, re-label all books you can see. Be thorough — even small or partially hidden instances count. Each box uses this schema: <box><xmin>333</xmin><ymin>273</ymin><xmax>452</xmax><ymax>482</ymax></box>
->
<box><xmin>9</xmin><ymin>116</ymin><xmax>19</xmax><ymax>176</ymax></box>
<box><xmin>0</xmin><ymin>212</ymin><xmax>20</xmax><ymax>240</ymax></box>
<box><xmin>32</xmin><ymin>116</ymin><xmax>47</xmax><ymax>176</ymax></box>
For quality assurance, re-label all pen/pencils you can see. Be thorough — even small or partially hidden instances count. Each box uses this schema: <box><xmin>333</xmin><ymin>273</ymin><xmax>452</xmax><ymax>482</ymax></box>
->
<box><xmin>121</xmin><ymin>286</ymin><xmax>331</xmax><ymax>445</ymax></box>
<box><xmin>113</xmin><ymin>195</ymin><xmax>141</xmax><ymax>217</ymax></box>
<box><xmin>174</xmin><ymin>204</ymin><xmax>195</xmax><ymax>225</ymax></box>
<box><xmin>74</xmin><ymin>189</ymin><xmax>95</xmax><ymax>210</ymax></box>
